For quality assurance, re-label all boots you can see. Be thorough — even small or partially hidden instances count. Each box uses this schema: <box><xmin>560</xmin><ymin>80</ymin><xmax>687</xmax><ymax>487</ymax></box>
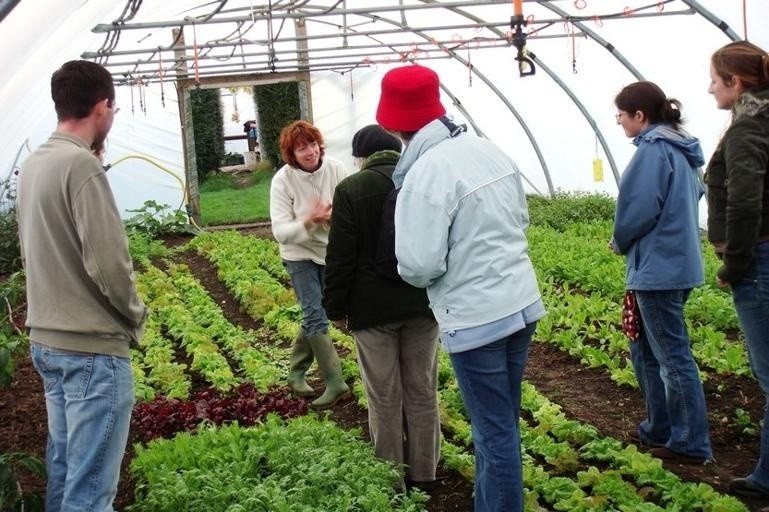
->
<box><xmin>287</xmin><ymin>327</ymin><xmax>316</xmax><ymax>397</ymax></box>
<box><xmin>307</xmin><ymin>333</ymin><xmax>352</xmax><ymax>411</ymax></box>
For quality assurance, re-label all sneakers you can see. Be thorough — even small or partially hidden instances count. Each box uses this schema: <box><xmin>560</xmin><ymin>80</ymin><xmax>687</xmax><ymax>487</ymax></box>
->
<box><xmin>626</xmin><ymin>428</ymin><xmax>642</xmax><ymax>441</ymax></box>
<box><xmin>647</xmin><ymin>446</ymin><xmax>707</xmax><ymax>465</ymax></box>
<box><xmin>730</xmin><ymin>477</ymin><xmax>765</xmax><ymax>497</ymax></box>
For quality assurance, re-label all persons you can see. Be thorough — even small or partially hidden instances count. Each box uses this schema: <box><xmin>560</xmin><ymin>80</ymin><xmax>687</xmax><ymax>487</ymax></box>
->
<box><xmin>268</xmin><ymin>120</ymin><xmax>349</xmax><ymax>411</ymax></box>
<box><xmin>374</xmin><ymin>64</ymin><xmax>548</xmax><ymax>512</ymax></box>
<box><xmin>703</xmin><ymin>41</ymin><xmax>768</xmax><ymax>510</ymax></box>
<box><xmin>321</xmin><ymin>125</ymin><xmax>440</xmax><ymax>498</ymax></box>
<box><xmin>12</xmin><ymin>59</ymin><xmax>150</xmax><ymax>512</ymax></box>
<box><xmin>606</xmin><ymin>79</ymin><xmax>713</xmax><ymax>465</ymax></box>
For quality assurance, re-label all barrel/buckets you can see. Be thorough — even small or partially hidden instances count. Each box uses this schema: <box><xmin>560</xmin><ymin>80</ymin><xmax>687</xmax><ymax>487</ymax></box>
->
<box><xmin>243</xmin><ymin>150</ymin><xmax>257</xmax><ymax>170</ymax></box>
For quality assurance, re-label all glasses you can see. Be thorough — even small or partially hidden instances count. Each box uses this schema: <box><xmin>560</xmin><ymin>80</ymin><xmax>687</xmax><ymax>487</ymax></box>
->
<box><xmin>616</xmin><ymin>112</ymin><xmax>629</xmax><ymax>120</ymax></box>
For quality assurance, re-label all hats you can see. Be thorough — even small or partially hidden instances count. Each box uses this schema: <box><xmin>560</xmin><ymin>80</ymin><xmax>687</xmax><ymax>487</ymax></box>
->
<box><xmin>376</xmin><ymin>64</ymin><xmax>447</xmax><ymax>133</ymax></box>
<box><xmin>351</xmin><ymin>124</ymin><xmax>403</xmax><ymax>159</ymax></box>
